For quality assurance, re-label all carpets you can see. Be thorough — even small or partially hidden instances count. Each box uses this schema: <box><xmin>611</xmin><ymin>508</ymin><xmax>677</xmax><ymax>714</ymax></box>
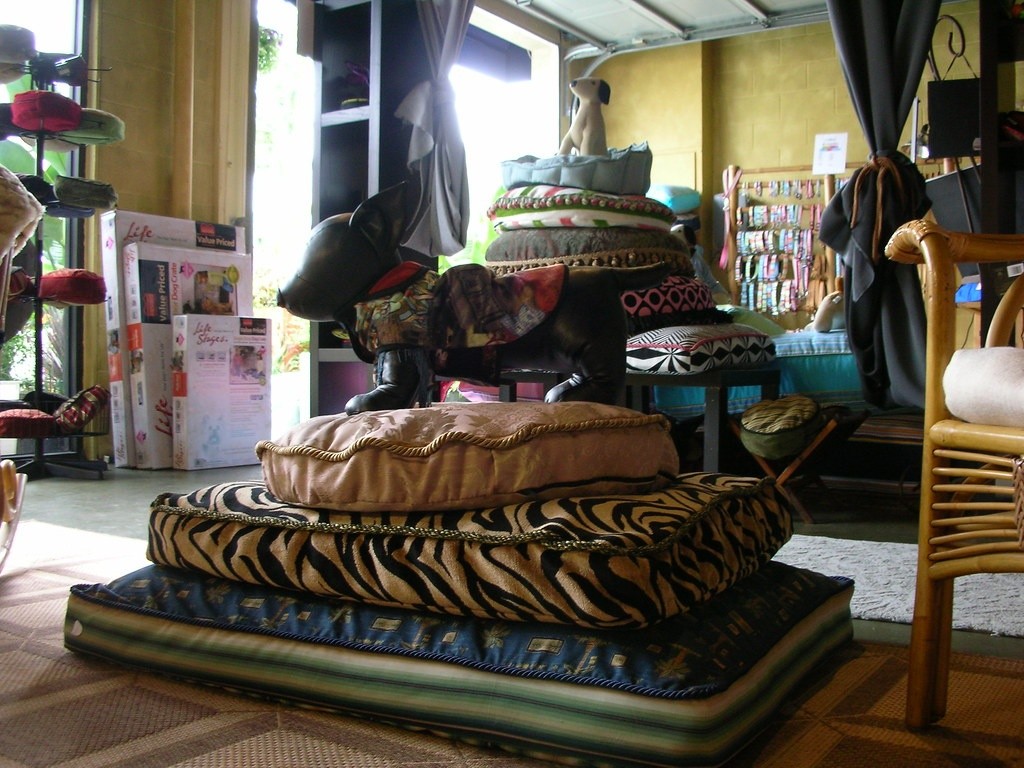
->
<box><xmin>2</xmin><ymin>516</ymin><xmax>1024</xmax><ymax>768</ymax></box>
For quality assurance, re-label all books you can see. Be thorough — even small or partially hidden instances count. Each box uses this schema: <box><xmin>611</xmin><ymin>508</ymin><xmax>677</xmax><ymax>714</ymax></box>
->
<box><xmin>171</xmin><ymin>314</ymin><xmax>273</xmax><ymax>472</ymax></box>
<box><xmin>123</xmin><ymin>241</ymin><xmax>254</xmax><ymax>470</ymax></box>
<box><xmin>101</xmin><ymin>210</ymin><xmax>246</xmax><ymax>468</ymax></box>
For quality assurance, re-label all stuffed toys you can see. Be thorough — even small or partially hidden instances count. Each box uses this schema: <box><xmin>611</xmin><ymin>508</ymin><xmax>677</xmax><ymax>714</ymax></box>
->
<box><xmin>558</xmin><ymin>77</ymin><xmax>612</xmax><ymax>156</ymax></box>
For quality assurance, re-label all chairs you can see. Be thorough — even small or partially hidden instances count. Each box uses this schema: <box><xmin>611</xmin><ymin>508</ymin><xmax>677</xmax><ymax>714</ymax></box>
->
<box><xmin>884</xmin><ymin>220</ymin><xmax>1024</xmax><ymax>729</ymax></box>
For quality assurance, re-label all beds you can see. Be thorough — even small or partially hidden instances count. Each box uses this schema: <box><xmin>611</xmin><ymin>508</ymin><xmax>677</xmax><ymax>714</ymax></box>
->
<box><xmin>649</xmin><ymin>328</ymin><xmax>864</xmax><ymax>423</ymax></box>
